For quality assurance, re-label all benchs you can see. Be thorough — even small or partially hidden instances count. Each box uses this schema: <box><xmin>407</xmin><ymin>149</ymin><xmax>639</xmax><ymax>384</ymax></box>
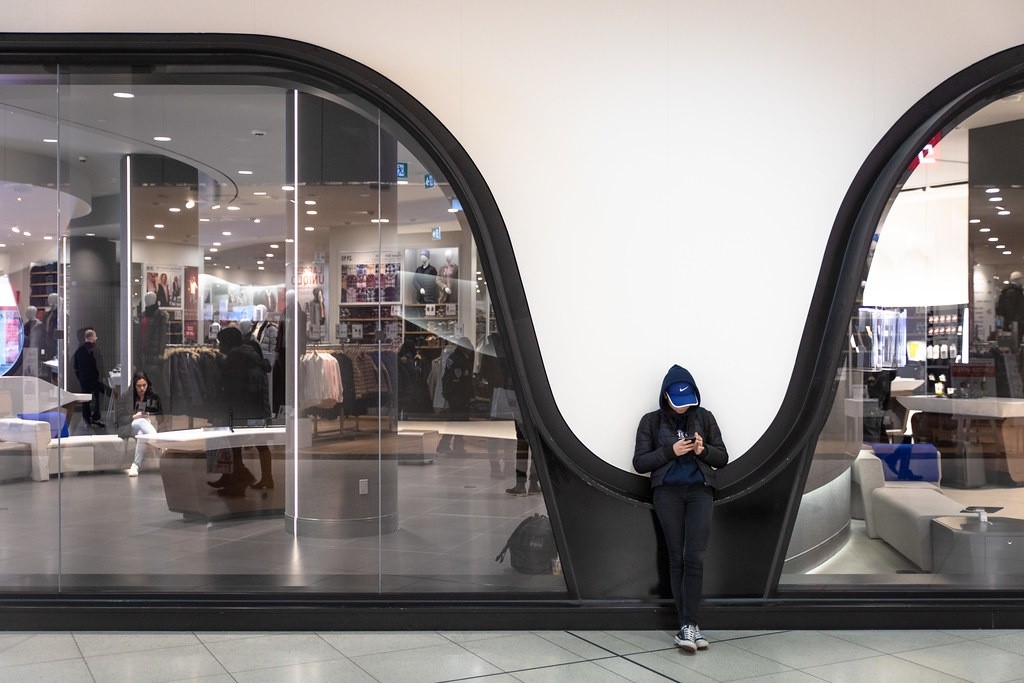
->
<box><xmin>0</xmin><ymin>418</ymin><xmax>163</xmax><ymax>481</ymax></box>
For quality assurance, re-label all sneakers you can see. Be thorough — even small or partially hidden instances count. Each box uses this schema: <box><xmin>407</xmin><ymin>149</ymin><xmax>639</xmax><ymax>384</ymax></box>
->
<box><xmin>674</xmin><ymin>625</ymin><xmax>697</xmax><ymax>653</ymax></box>
<box><xmin>694</xmin><ymin>626</ymin><xmax>709</xmax><ymax>647</ymax></box>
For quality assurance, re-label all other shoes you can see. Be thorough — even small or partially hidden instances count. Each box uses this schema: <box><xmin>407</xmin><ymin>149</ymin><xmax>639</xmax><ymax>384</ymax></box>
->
<box><xmin>898</xmin><ymin>469</ymin><xmax>924</xmax><ymax>481</ymax></box>
<box><xmin>436</xmin><ymin>440</ymin><xmax>453</xmax><ymax>456</ymax></box>
<box><xmin>528</xmin><ymin>484</ymin><xmax>543</xmax><ymax>495</ymax></box>
<box><xmin>205</xmin><ymin>465</ymin><xmax>275</xmax><ymax>496</ymax></box>
<box><xmin>128</xmin><ymin>463</ymin><xmax>139</xmax><ymax>476</ymax></box>
<box><xmin>884</xmin><ymin>453</ymin><xmax>898</xmax><ymax>475</ymax></box>
<box><xmin>92</xmin><ymin>418</ymin><xmax>106</xmax><ymax>428</ymax></box>
<box><xmin>490</xmin><ymin>467</ymin><xmax>506</xmax><ymax>480</ymax></box>
<box><xmin>505</xmin><ymin>483</ymin><xmax>527</xmax><ymax>496</ymax></box>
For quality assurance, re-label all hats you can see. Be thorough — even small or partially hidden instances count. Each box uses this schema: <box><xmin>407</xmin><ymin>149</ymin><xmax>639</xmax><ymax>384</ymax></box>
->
<box><xmin>420</xmin><ymin>250</ymin><xmax>430</xmax><ymax>259</ymax></box>
<box><xmin>665</xmin><ymin>381</ymin><xmax>699</xmax><ymax>408</ymax></box>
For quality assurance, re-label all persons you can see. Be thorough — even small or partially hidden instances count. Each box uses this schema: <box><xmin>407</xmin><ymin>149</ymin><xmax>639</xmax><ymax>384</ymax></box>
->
<box><xmin>136</xmin><ymin>292</ymin><xmax>169</xmax><ymax>374</ymax></box>
<box><xmin>412</xmin><ymin>249</ymin><xmax>438</xmax><ymax>303</ymax></box>
<box><xmin>73</xmin><ymin>330</ymin><xmax>106</xmax><ymax>429</ymax></box>
<box><xmin>205</xmin><ymin>320</ymin><xmax>276</xmax><ymax>499</ymax></box>
<box><xmin>437</xmin><ymin>250</ymin><xmax>458</xmax><ymax>303</ymax></box>
<box><xmin>273</xmin><ymin>290</ymin><xmax>305</xmax><ymax>418</ymax></box>
<box><xmin>146</xmin><ymin>272</ymin><xmax>198</xmax><ymax>306</ymax></box>
<box><xmin>632</xmin><ymin>364</ymin><xmax>729</xmax><ymax>651</ymax></box>
<box><xmin>861</xmin><ymin>386</ymin><xmax>924</xmax><ymax>483</ymax></box>
<box><xmin>397</xmin><ymin>332</ymin><xmax>542</xmax><ymax>497</ymax></box>
<box><xmin>21</xmin><ymin>293</ymin><xmax>63</xmax><ymax>363</ymax></box>
<box><xmin>309</xmin><ymin>288</ymin><xmax>326</xmax><ymax>325</ymax></box>
<box><xmin>996</xmin><ymin>271</ymin><xmax>1024</xmax><ymax>354</ymax></box>
<box><xmin>115</xmin><ymin>371</ymin><xmax>163</xmax><ymax>477</ymax></box>
<box><xmin>238</xmin><ymin>305</ymin><xmax>277</xmax><ymax>361</ymax></box>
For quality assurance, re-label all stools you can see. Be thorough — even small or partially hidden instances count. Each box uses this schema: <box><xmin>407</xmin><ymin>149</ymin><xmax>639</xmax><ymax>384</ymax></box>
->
<box><xmin>872</xmin><ymin>487</ymin><xmax>980</xmax><ymax>574</ymax></box>
<box><xmin>396</xmin><ymin>429</ymin><xmax>439</xmax><ymax>465</ymax></box>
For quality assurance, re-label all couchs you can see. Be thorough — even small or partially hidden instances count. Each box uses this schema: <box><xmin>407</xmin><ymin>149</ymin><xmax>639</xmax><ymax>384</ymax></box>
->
<box><xmin>851</xmin><ymin>443</ymin><xmax>942</xmax><ymax>538</ymax></box>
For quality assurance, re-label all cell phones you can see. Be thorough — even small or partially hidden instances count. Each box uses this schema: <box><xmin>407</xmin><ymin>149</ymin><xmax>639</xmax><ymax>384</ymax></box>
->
<box><xmin>684</xmin><ymin>437</ymin><xmax>696</xmax><ymax>444</ymax></box>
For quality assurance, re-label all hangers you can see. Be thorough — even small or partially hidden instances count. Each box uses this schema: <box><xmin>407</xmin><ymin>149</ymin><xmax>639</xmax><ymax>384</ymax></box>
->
<box><xmin>415</xmin><ymin>351</ymin><xmax>421</xmax><ymax>360</ymax></box>
<box><xmin>160</xmin><ymin>344</ymin><xmax>219</xmax><ymax>359</ymax></box>
<box><xmin>304</xmin><ymin>345</ymin><xmax>393</xmax><ymax>355</ymax></box>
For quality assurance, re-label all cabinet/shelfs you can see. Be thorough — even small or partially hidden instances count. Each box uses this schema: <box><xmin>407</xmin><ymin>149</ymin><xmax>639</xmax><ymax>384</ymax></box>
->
<box><xmin>931</xmin><ymin>517</ymin><xmax>1024</xmax><ymax>578</ymax></box>
<box><xmin>340</xmin><ymin>301</ymin><xmax>403</xmax><ymax>345</ymax></box>
<box><xmin>159</xmin><ymin>306</ymin><xmax>182</xmax><ymax>337</ymax></box>
<box><xmin>402</xmin><ymin>247</ymin><xmax>460</xmax><ymax>348</ymax></box>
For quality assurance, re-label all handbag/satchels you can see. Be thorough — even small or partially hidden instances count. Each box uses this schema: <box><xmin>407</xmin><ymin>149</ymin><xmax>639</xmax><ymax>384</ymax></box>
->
<box><xmin>205</xmin><ymin>436</ymin><xmax>234</xmax><ymax>474</ymax></box>
<box><xmin>490</xmin><ymin>387</ymin><xmax>524</xmax><ymax>423</ymax></box>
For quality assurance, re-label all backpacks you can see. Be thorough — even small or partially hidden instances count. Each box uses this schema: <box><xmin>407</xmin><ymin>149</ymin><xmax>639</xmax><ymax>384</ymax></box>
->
<box><xmin>495</xmin><ymin>513</ymin><xmax>556</xmax><ymax>574</ymax></box>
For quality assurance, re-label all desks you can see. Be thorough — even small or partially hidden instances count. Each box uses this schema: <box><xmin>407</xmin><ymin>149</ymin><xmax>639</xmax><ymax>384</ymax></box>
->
<box><xmin>0</xmin><ymin>376</ymin><xmax>92</xmax><ymax>434</ymax></box>
<box><xmin>134</xmin><ymin>427</ymin><xmax>287</xmax><ymax>522</ymax></box>
<box><xmin>896</xmin><ymin>396</ymin><xmax>1024</xmax><ymax>486</ymax></box>
